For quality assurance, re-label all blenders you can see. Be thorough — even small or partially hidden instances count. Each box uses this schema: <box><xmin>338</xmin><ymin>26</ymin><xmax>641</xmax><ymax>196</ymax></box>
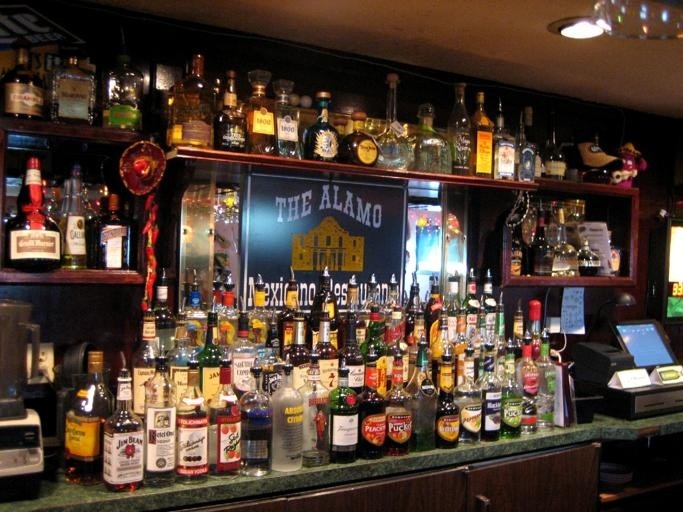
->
<box><xmin>1</xmin><ymin>299</ymin><xmax>47</xmax><ymax>503</ymax></box>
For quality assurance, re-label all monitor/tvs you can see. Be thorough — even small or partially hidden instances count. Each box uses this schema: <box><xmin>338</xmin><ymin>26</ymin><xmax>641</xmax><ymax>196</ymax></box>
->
<box><xmin>608</xmin><ymin>318</ymin><xmax>680</xmax><ymax>369</ymax></box>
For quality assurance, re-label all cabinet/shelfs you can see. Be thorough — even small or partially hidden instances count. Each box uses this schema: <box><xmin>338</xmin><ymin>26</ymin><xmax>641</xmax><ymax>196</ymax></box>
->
<box><xmin>0</xmin><ymin>114</ymin><xmax>149</xmax><ymax>286</ymax></box>
<box><xmin>160</xmin><ymin>441</ymin><xmax>601</xmax><ymax>512</ymax></box>
<box><xmin>601</xmin><ymin>422</ymin><xmax>683</xmax><ymax>504</ymax></box>
<box><xmin>490</xmin><ymin>175</ymin><xmax>640</xmax><ymax>287</ymax></box>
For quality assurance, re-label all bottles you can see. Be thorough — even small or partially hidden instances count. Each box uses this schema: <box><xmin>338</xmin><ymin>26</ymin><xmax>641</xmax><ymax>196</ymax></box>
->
<box><xmin>248</xmin><ymin>70</ymin><xmax>278</xmax><ymax>156</ymax></box>
<box><xmin>104</xmin><ymin>263</ymin><xmax>557</xmax><ymax>494</ymax></box>
<box><xmin>62</xmin><ymin>349</ymin><xmax>114</xmax><ymax>488</ymax></box>
<box><xmin>99</xmin><ymin>52</ymin><xmax>146</xmax><ymax>133</ymax></box>
<box><xmin>475</xmin><ymin>90</ymin><xmax>495</xmax><ymax>179</ymax></box>
<box><xmin>516</xmin><ymin>106</ymin><xmax>611</xmax><ymax>185</ymax></box>
<box><xmin>380</xmin><ymin>72</ymin><xmax>416</xmax><ymax>171</ymax></box>
<box><xmin>277</xmin><ymin>78</ymin><xmax>301</xmax><ymax>156</ymax></box>
<box><xmin>494</xmin><ymin>97</ymin><xmax>516</xmax><ymax>181</ymax></box>
<box><xmin>216</xmin><ymin>70</ymin><xmax>248</xmax><ymax>152</ymax></box>
<box><xmin>507</xmin><ymin>188</ymin><xmax>623</xmax><ymax>277</ymax></box>
<box><xmin>3</xmin><ymin>45</ymin><xmax>48</xmax><ymax>120</ymax></box>
<box><xmin>416</xmin><ymin>104</ymin><xmax>446</xmax><ymax>173</ymax></box>
<box><xmin>345</xmin><ymin>110</ymin><xmax>380</xmax><ymax>165</ymax></box>
<box><xmin>4</xmin><ymin>155</ymin><xmax>137</xmax><ymax>272</ymax></box>
<box><xmin>50</xmin><ymin>39</ymin><xmax>98</xmax><ymax>124</ymax></box>
<box><xmin>303</xmin><ymin>89</ymin><xmax>344</xmax><ymax>163</ymax></box>
<box><xmin>447</xmin><ymin>81</ymin><xmax>474</xmax><ymax>177</ymax></box>
<box><xmin>168</xmin><ymin>53</ymin><xmax>215</xmax><ymax>149</ymax></box>
